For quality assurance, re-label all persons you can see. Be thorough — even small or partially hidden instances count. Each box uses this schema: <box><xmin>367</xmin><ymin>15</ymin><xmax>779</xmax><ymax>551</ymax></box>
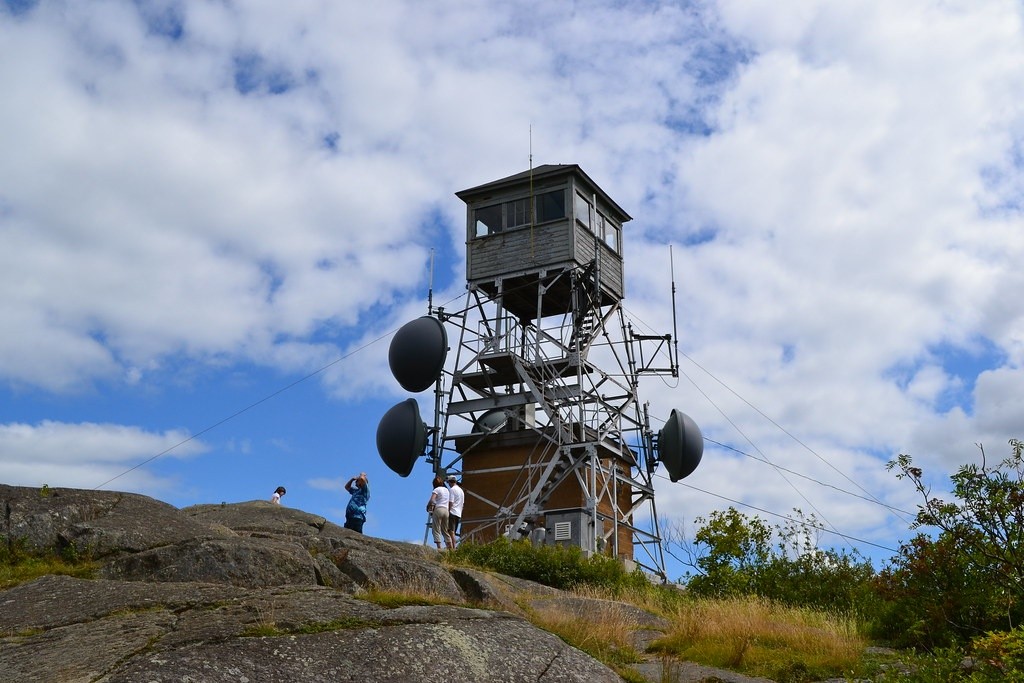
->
<box><xmin>271</xmin><ymin>487</ymin><xmax>287</xmax><ymax>505</ymax></box>
<box><xmin>428</xmin><ymin>476</ymin><xmax>464</xmax><ymax>550</ymax></box>
<box><xmin>344</xmin><ymin>472</ymin><xmax>370</xmax><ymax>534</ymax></box>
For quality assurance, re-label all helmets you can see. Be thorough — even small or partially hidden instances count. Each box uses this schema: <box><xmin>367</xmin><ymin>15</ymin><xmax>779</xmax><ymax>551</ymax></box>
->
<box><xmin>447</xmin><ymin>475</ymin><xmax>456</xmax><ymax>482</ymax></box>
